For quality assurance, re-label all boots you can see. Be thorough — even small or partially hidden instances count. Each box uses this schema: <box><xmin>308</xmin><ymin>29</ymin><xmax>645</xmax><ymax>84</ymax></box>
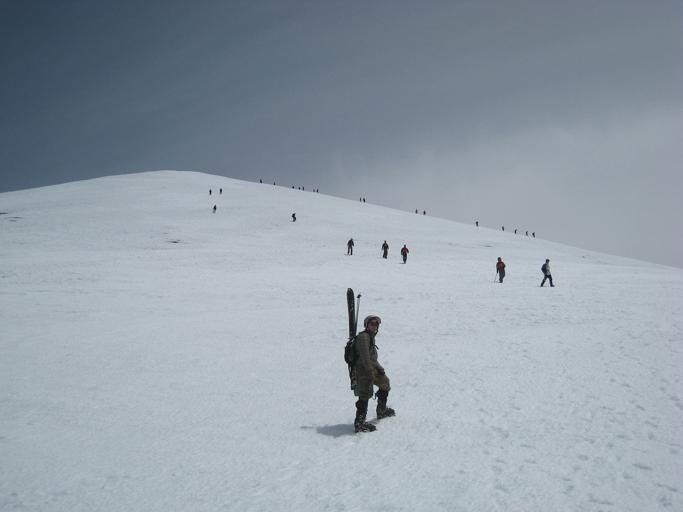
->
<box><xmin>354</xmin><ymin>412</ymin><xmax>376</xmax><ymax>432</ymax></box>
<box><xmin>376</xmin><ymin>404</ymin><xmax>394</xmax><ymax>419</ymax></box>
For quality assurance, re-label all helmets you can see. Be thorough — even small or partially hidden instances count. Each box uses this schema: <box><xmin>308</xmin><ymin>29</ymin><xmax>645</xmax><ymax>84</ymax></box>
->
<box><xmin>363</xmin><ymin>315</ymin><xmax>381</xmax><ymax>327</ymax></box>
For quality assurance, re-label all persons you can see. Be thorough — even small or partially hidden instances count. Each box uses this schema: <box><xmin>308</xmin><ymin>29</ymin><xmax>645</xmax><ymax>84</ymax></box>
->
<box><xmin>501</xmin><ymin>224</ymin><xmax>504</xmax><ymax>231</ymax></box>
<box><xmin>514</xmin><ymin>228</ymin><xmax>517</xmax><ymax>234</ymax></box>
<box><xmin>259</xmin><ymin>179</ymin><xmax>318</xmax><ymax>192</ymax></box>
<box><xmin>291</xmin><ymin>211</ymin><xmax>296</xmax><ymax>222</ymax></box>
<box><xmin>347</xmin><ymin>238</ymin><xmax>354</xmax><ymax>256</ymax></box>
<box><xmin>208</xmin><ymin>189</ymin><xmax>212</xmax><ymax>195</ymax></box>
<box><xmin>219</xmin><ymin>188</ymin><xmax>222</xmax><ymax>194</ymax></box>
<box><xmin>532</xmin><ymin>232</ymin><xmax>536</xmax><ymax>238</ymax></box>
<box><xmin>475</xmin><ymin>220</ymin><xmax>479</xmax><ymax>227</ymax></box>
<box><xmin>415</xmin><ymin>208</ymin><xmax>418</xmax><ymax>214</ymax></box>
<box><xmin>495</xmin><ymin>257</ymin><xmax>506</xmax><ymax>283</ymax></box>
<box><xmin>349</xmin><ymin>314</ymin><xmax>397</xmax><ymax>433</ymax></box>
<box><xmin>381</xmin><ymin>240</ymin><xmax>389</xmax><ymax>259</ymax></box>
<box><xmin>359</xmin><ymin>197</ymin><xmax>362</xmax><ymax>202</ymax></box>
<box><xmin>362</xmin><ymin>197</ymin><xmax>365</xmax><ymax>203</ymax></box>
<box><xmin>539</xmin><ymin>258</ymin><xmax>556</xmax><ymax>287</ymax></box>
<box><xmin>212</xmin><ymin>204</ymin><xmax>217</xmax><ymax>213</ymax></box>
<box><xmin>525</xmin><ymin>231</ymin><xmax>528</xmax><ymax>236</ymax></box>
<box><xmin>423</xmin><ymin>210</ymin><xmax>426</xmax><ymax>214</ymax></box>
<box><xmin>399</xmin><ymin>244</ymin><xmax>409</xmax><ymax>264</ymax></box>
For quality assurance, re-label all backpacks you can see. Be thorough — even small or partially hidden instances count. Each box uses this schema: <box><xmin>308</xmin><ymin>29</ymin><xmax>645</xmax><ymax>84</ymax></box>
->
<box><xmin>343</xmin><ymin>330</ymin><xmax>372</xmax><ymax>365</ymax></box>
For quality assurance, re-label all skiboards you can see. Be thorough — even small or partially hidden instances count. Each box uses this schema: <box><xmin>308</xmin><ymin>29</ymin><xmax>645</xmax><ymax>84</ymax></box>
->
<box><xmin>347</xmin><ymin>288</ymin><xmax>356</xmax><ymax>390</ymax></box>
